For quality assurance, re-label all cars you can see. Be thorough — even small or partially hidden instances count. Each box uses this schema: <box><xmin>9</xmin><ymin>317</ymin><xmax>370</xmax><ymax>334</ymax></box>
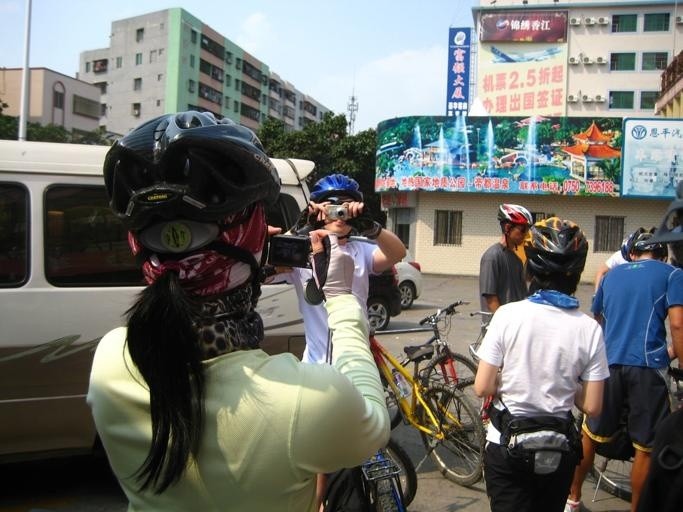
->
<box><xmin>367</xmin><ymin>248</ymin><xmax>423</xmax><ymax>331</ymax></box>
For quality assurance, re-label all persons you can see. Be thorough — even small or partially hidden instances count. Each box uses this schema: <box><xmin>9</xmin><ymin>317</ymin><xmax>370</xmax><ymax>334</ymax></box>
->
<box><xmin>264</xmin><ymin>170</ymin><xmax>407</xmax><ymax>512</ymax></box>
<box><xmin>480</xmin><ymin>201</ymin><xmax>532</xmax><ymax>335</ymax></box>
<box><xmin>86</xmin><ymin>109</ymin><xmax>392</xmax><ymax>512</ymax></box>
<box><xmin>473</xmin><ymin>217</ymin><xmax>613</xmax><ymax>512</ymax></box>
<box><xmin>561</xmin><ymin>233</ymin><xmax>683</xmax><ymax>511</ymax></box>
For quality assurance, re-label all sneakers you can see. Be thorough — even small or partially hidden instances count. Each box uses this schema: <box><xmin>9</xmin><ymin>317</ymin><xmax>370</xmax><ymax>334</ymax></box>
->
<box><xmin>563</xmin><ymin>499</ymin><xmax>581</xmax><ymax>512</ymax></box>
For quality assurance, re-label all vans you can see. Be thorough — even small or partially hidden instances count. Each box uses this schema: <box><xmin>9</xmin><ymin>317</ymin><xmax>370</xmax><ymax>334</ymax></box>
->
<box><xmin>0</xmin><ymin>139</ymin><xmax>315</xmax><ymax>499</ymax></box>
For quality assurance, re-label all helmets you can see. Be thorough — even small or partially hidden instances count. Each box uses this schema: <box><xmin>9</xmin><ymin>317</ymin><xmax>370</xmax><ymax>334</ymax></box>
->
<box><xmin>521</xmin><ymin>217</ymin><xmax>591</xmax><ymax>273</ymax></box>
<box><xmin>496</xmin><ymin>203</ymin><xmax>532</xmax><ymax>226</ymax></box>
<box><xmin>101</xmin><ymin>111</ymin><xmax>285</xmax><ymax>226</ymax></box>
<box><xmin>619</xmin><ymin>226</ymin><xmax>667</xmax><ymax>261</ymax></box>
<box><xmin>310</xmin><ymin>173</ymin><xmax>365</xmax><ymax>203</ymax></box>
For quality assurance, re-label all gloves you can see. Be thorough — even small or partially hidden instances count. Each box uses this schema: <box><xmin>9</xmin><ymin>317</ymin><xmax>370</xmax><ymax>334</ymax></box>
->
<box><xmin>342</xmin><ymin>204</ymin><xmax>372</xmax><ymax>236</ymax></box>
<box><xmin>308</xmin><ymin>232</ymin><xmax>356</xmax><ymax>303</ymax></box>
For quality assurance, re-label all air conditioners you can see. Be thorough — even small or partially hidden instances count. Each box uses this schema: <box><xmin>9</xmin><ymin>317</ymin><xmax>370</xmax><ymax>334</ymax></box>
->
<box><xmin>567</xmin><ymin>16</ymin><xmax>609</xmax><ymax>103</ymax></box>
<box><xmin>675</xmin><ymin>16</ymin><xmax>683</xmax><ymax>24</ymax></box>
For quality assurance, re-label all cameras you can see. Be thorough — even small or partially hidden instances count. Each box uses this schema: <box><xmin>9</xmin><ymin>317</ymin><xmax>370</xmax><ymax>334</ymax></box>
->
<box><xmin>326</xmin><ymin>204</ymin><xmax>350</xmax><ymax>222</ymax></box>
<box><xmin>266</xmin><ymin>233</ymin><xmax>319</xmax><ymax>269</ymax></box>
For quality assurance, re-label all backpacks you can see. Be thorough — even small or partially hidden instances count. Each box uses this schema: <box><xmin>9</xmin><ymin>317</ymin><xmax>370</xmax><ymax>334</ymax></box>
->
<box><xmin>496</xmin><ymin>409</ymin><xmax>581</xmax><ymax>474</ymax></box>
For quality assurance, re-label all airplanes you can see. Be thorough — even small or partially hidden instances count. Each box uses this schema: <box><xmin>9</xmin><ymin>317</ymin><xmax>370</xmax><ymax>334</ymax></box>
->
<box><xmin>489</xmin><ymin>43</ymin><xmax>562</xmax><ymax>64</ymax></box>
